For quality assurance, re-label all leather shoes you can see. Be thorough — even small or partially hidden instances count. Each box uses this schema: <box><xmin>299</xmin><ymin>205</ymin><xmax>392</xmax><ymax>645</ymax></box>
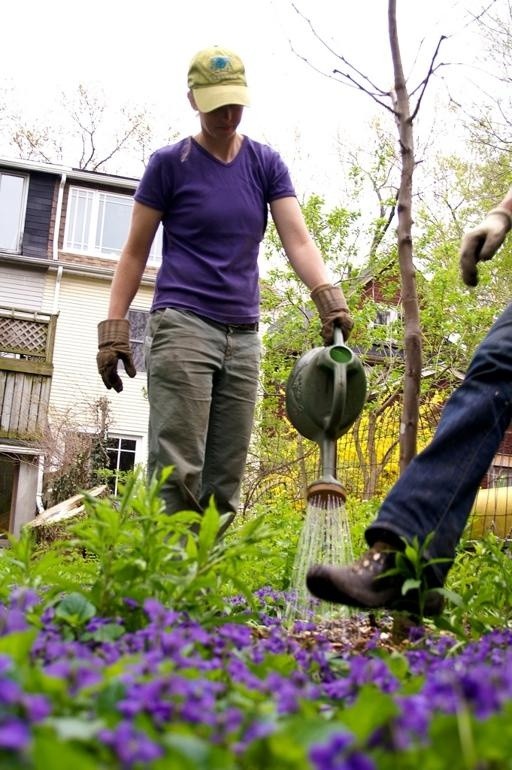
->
<box><xmin>306</xmin><ymin>551</ymin><xmax>443</xmax><ymax>617</ymax></box>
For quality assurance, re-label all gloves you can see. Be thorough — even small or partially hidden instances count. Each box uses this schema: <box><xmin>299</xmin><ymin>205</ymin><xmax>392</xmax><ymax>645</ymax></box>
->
<box><xmin>459</xmin><ymin>208</ymin><xmax>512</xmax><ymax>287</ymax></box>
<box><xmin>96</xmin><ymin>319</ymin><xmax>136</xmax><ymax>392</ymax></box>
<box><xmin>310</xmin><ymin>283</ymin><xmax>355</xmax><ymax>347</ymax></box>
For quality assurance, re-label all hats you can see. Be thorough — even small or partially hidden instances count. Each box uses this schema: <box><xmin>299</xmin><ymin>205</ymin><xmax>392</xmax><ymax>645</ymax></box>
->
<box><xmin>187</xmin><ymin>45</ymin><xmax>252</xmax><ymax>114</ymax></box>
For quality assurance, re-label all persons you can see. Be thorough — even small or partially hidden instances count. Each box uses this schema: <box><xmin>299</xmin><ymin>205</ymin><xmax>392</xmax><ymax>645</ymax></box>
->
<box><xmin>309</xmin><ymin>151</ymin><xmax>512</xmax><ymax>614</ymax></box>
<box><xmin>95</xmin><ymin>48</ymin><xmax>346</xmax><ymax>533</ymax></box>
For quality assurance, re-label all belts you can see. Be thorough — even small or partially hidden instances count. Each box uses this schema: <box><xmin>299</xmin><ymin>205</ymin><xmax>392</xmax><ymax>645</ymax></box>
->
<box><xmin>228</xmin><ymin>322</ymin><xmax>259</xmax><ymax>331</ymax></box>
